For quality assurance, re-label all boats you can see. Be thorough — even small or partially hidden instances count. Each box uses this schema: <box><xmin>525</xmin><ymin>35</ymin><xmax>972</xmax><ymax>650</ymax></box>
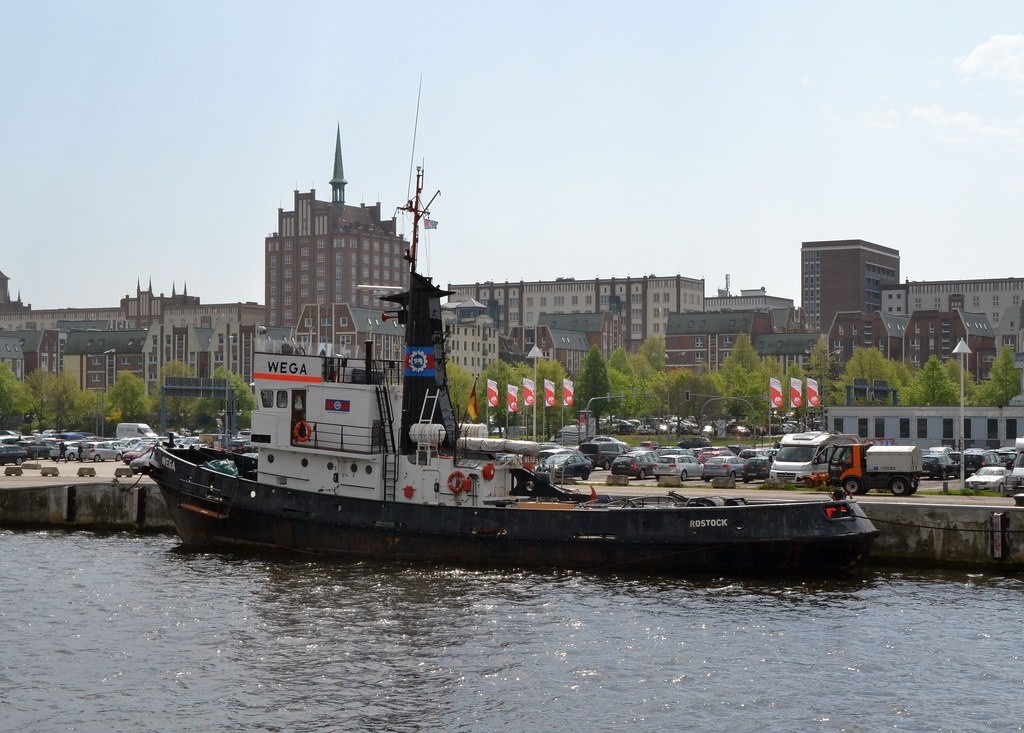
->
<box><xmin>149</xmin><ymin>74</ymin><xmax>882</xmax><ymax>562</ymax></box>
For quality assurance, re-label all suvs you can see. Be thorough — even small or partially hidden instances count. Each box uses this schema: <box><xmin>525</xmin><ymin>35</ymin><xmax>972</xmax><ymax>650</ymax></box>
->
<box><xmin>963</xmin><ymin>452</ymin><xmax>1007</xmax><ymax>477</ymax></box>
<box><xmin>579</xmin><ymin>442</ymin><xmax>627</xmax><ymax>471</ymax></box>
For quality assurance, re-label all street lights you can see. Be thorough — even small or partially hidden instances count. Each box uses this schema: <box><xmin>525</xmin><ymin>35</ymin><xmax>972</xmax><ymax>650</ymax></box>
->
<box><xmin>225</xmin><ymin>334</ymin><xmax>234</xmax><ymax>448</ymax></box>
<box><xmin>100</xmin><ymin>349</ymin><xmax>116</xmax><ymax>437</ymax></box>
<box><xmin>527</xmin><ymin>325</ymin><xmax>543</xmax><ymax>442</ymax></box>
<box><xmin>821</xmin><ymin>349</ymin><xmax>842</xmax><ymax>432</ymax></box>
<box><xmin>664</xmin><ymin>351</ymin><xmax>685</xmax><ymax>446</ymax></box>
<box><xmin>952</xmin><ymin>338</ymin><xmax>972</xmax><ymax>490</ymax></box>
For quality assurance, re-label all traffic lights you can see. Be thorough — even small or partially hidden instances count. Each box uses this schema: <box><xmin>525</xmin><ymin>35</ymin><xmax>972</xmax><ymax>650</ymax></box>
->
<box><xmin>684</xmin><ymin>391</ymin><xmax>690</xmax><ymax>401</ymax></box>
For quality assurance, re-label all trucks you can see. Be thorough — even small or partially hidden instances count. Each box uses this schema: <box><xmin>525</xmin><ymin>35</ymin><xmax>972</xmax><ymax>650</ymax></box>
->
<box><xmin>826</xmin><ymin>443</ymin><xmax>922</xmax><ymax>497</ymax></box>
<box><xmin>1011</xmin><ymin>438</ymin><xmax>1024</xmax><ymax>488</ymax></box>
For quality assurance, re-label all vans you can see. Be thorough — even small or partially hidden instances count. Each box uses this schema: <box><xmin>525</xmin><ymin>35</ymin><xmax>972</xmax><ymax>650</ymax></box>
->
<box><xmin>769</xmin><ymin>432</ymin><xmax>860</xmax><ymax>490</ymax></box>
<box><xmin>83</xmin><ymin>442</ymin><xmax>122</xmax><ymax>462</ymax></box>
<box><xmin>115</xmin><ymin>423</ymin><xmax>157</xmax><ymax>437</ymax></box>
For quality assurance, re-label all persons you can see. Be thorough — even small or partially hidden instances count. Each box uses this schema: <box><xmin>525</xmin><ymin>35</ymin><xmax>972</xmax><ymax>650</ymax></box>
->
<box><xmin>56</xmin><ymin>440</ymin><xmax>67</xmax><ymax>463</ymax></box>
<box><xmin>78</xmin><ymin>441</ymin><xmax>83</xmax><ymax>462</ymax></box>
<box><xmin>17</xmin><ymin>436</ymin><xmax>24</xmax><ymax>441</ymax></box>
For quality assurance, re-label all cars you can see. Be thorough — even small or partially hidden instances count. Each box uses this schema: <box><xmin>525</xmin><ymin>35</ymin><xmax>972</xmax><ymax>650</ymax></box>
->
<box><xmin>920</xmin><ymin>446</ymin><xmax>1017</xmax><ymax>466</ymax></box>
<box><xmin>652</xmin><ymin>455</ymin><xmax>705</xmax><ymax>482</ymax></box>
<box><xmin>538</xmin><ymin>416</ymin><xmax>811</xmax><ymax>465</ymax></box>
<box><xmin>922</xmin><ymin>455</ymin><xmax>960</xmax><ymax>479</ymax></box>
<box><xmin>610</xmin><ymin>453</ymin><xmax>659</xmax><ymax>480</ymax></box>
<box><xmin>703</xmin><ymin>456</ymin><xmax>745</xmax><ymax>481</ymax></box>
<box><xmin>965</xmin><ymin>466</ymin><xmax>1010</xmax><ymax>493</ymax></box>
<box><xmin>535</xmin><ymin>454</ymin><xmax>592</xmax><ymax>483</ymax></box>
<box><xmin>0</xmin><ymin>428</ymin><xmax>252</xmax><ymax>472</ymax></box>
<box><xmin>743</xmin><ymin>457</ymin><xmax>773</xmax><ymax>483</ymax></box>
<box><xmin>676</xmin><ymin>437</ymin><xmax>714</xmax><ymax>449</ymax></box>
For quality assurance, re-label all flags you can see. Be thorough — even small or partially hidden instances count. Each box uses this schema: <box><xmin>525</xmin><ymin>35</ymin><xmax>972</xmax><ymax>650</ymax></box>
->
<box><xmin>424</xmin><ymin>219</ymin><xmax>438</xmax><ymax>230</ymax></box>
<box><xmin>770</xmin><ymin>378</ymin><xmax>783</xmax><ymax>407</ymax></box>
<box><xmin>791</xmin><ymin>378</ymin><xmax>803</xmax><ymax>407</ymax></box>
<box><xmin>487</xmin><ymin>379</ymin><xmax>498</xmax><ymax>406</ymax></box>
<box><xmin>523</xmin><ymin>378</ymin><xmax>534</xmax><ymax>406</ymax></box>
<box><xmin>564</xmin><ymin>379</ymin><xmax>574</xmax><ymax>405</ymax></box>
<box><xmin>508</xmin><ymin>385</ymin><xmax>519</xmax><ymax>412</ymax></box>
<box><xmin>807</xmin><ymin>378</ymin><xmax>819</xmax><ymax>406</ymax></box>
<box><xmin>545</xmin><ymin>379</ymin><xmax>555</xmax><ymax>406</ymax></box>
<box><xmin>467</xmin><ymin>385</ymin><xmax>478</xmax><ymax>421</ymax></box>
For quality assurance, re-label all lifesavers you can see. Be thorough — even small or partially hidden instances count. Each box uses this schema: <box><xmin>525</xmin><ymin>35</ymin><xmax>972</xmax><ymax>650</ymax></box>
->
<box><xmin>448</xmin><ymin>471</ymin><xmax>465</xmax><ymax>492</ymax></box>
<box><xmin>294</xmin><ymin>421</ymin><xmax>312</xmax><ymax>442</ymax></box>
